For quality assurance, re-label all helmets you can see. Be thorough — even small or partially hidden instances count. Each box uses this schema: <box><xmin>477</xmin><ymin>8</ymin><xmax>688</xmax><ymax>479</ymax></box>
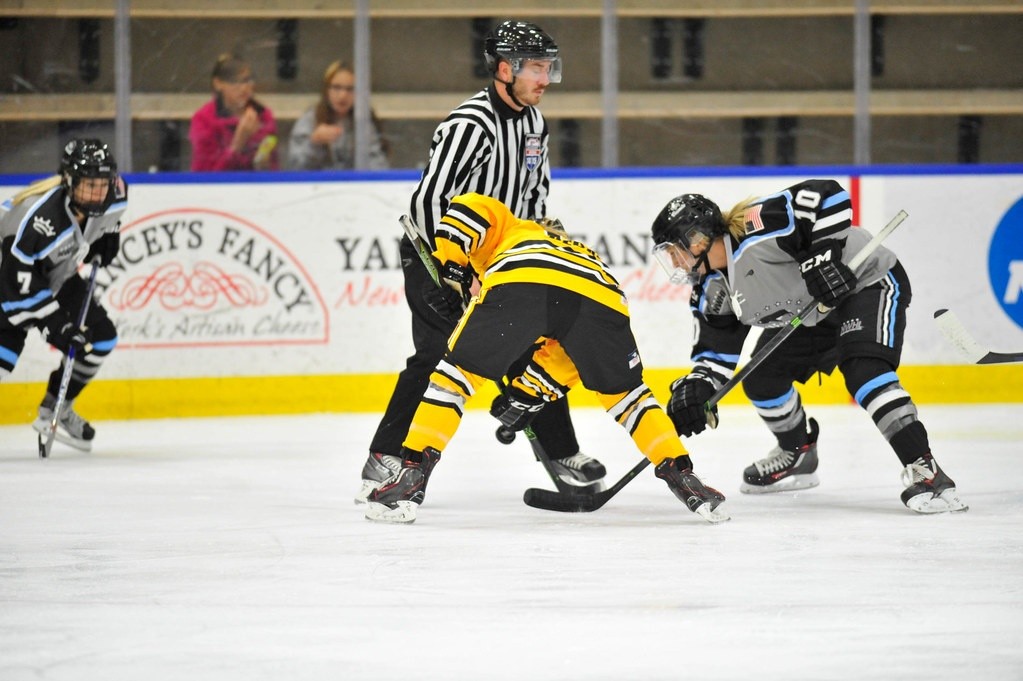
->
<box><xmin>58</xmin><ymin>137</ymin><xmax>116</xmax><ymax>178</ymax></box>
<box><xmin>483</xmin><ymin>19</ymin><xmax>559</xmax><ymax>70</ymax></box>
<box><xmin>535</xmin><ymin>218</ymin><xmax>565</xmax><ymax>232</ymax></box>
<box><xmin>651</xmin><ymin>194</ymin><xmax>725</xmax><ymax>251</ymax></box>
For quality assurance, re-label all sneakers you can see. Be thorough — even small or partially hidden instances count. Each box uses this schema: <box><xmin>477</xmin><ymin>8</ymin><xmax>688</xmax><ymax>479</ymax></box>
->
<box><xmin>34</xmin><ymin>393</ymin><xmax>95</xmax><ymax>452</ymax></box>
<box><xmin>353</xmin><ymin>446</ymin><xmax>403</xmax><ymax>504</ymax></box>
<box><xmin>899</xmin><ymin>454</ymin><xmax>969</xmax><ymax>514</ymax></box>
<box><xmin>551</xmin><ymin>452</ymin><xmax>607</xmax><ymax>487</ymax></box>
<box><xmin>654</xmin><ymin>456</ymin><xmax>731</xmax><ymax>524</ymax></box>
<box><xmin>740</xmin><ymin>417</ymin><xmax>820</xmax><ymax>496</ymax></box>
<box><xmin>365</xmin><ymin>445</ymin><xmax>441</xmax><ymax>525</ymax></box>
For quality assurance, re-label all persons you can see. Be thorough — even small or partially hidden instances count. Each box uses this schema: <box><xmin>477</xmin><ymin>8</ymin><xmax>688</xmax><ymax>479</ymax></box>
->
<box><xmin>0</xmin><ymin>138</ymin><xmax>126</xmax><ymax>453</ymax></box>
<box><xmin>189</xmin><ymin>51</ymin><xmax>280</xmax><ymax>171</ymax></box>
<box><xmin>363</xmin><ymin>192</ymin><xmax>742</xmax><ymax>524</ymax></box>
<box><xmin>361</xmin><ymin>21</ymin><xmax>606</xmax><ymax>494</ymax></box>
<box><xmin>652</xmin><ymin>179</ymin><xmax>967</xmax><ymax>513</ymax></box>
<box><xmin>288</xmin><ymin>61</ymin><xmax>393</xmax><ymax>171</ymax></box>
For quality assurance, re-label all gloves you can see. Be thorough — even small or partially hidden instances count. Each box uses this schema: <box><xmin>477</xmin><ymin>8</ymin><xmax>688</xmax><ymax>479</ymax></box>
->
<box><xmin>47</xmin><ymin>318</ymin><xmax>94</xmax><ymax>361</ymax></box>
<box><xmin>490</xmin><ymin>383</ymin><xmax>545</xmax><ymax>431</ymax></box>
<box><xmin>83</xmin><ymin>233</ymin><xmax>119</xmax><ymax>268</ymax></box>
<box><xmin>666</xmin><ymin>366</ymin><xmax>720</xmax><ymax>438</ymax></box>
<box><xmin>421</xmin><ymin>260</ymin><xmax>473</xmax><ymax>328</ymax></box>
<box><xmin>798</xmin><ymin>238</ymin><xmax>858</xmax><ymax>313</ymax></box>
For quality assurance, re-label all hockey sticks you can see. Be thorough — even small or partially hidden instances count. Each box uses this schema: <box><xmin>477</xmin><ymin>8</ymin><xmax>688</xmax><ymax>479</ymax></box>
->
<box><xmin>398</xmin><ymin>211</ymin><xmax>605</xmax><ymax>497</ymax></box>
<box><xmin>521</xmin><ymin>208</ymin><xmax>912</xmax><ymax>514</ymax></box>
<box><xmin>36</xmin><ymin>253</ymin><xmax>102</xmax><ymax>459</ymax></box>
<box><xmin>933</xmin><ymin>305</ymin><xmax>1023</xmax><ymax>368</ymax></box>
